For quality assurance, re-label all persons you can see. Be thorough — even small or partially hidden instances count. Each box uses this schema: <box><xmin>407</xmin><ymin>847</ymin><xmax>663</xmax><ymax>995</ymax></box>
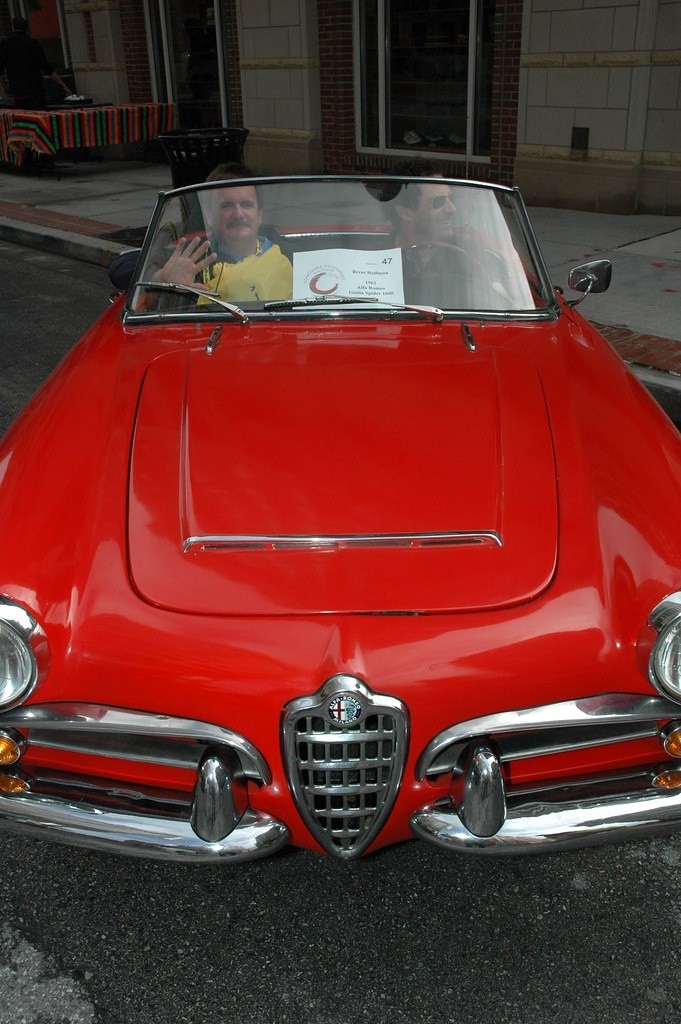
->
<box><xmin>108</xmin><ymin>162</ymin><xmax>301</xmax><ymax>311</ymax></box>
<box><xmin>0</xmin><ymin>16</ymin><xmax>53</xmax><ymax>110</ymax></box>
<box><xmin>372</xmin><ymin>156</ymin><xmax>524</xmax><ymax>309</ymax></box>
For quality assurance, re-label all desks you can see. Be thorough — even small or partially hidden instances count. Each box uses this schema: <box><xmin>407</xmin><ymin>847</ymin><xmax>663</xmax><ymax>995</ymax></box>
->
<box><xmin>48</xmin><ymin>99</ymin><xmax>113</xmax><ymax>110</ymax></box>
<box><xmin>0</xmin><ymin>101</ymin><xmax>181</xmax><ymax>179</ymax></box>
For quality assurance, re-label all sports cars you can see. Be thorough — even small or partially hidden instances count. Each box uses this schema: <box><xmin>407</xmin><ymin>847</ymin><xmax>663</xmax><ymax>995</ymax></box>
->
<box><xmin>0</xmin><ymin>174</ymin><xmax>681</xmax><ymax>868</ymax></box>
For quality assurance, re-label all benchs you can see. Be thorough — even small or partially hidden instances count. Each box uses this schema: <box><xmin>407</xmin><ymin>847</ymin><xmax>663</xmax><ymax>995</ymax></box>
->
<box><xmin>168</xmin><ymin>224</ymin><xmax>528</xmax><ymax>280</ymax></box>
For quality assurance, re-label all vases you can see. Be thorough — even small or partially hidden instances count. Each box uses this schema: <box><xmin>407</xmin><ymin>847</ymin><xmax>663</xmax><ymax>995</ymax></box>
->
<box><xmin>156</xmin><ymin>127</ymin><xmax>250</xmax><ymax>189</ymax></box>
